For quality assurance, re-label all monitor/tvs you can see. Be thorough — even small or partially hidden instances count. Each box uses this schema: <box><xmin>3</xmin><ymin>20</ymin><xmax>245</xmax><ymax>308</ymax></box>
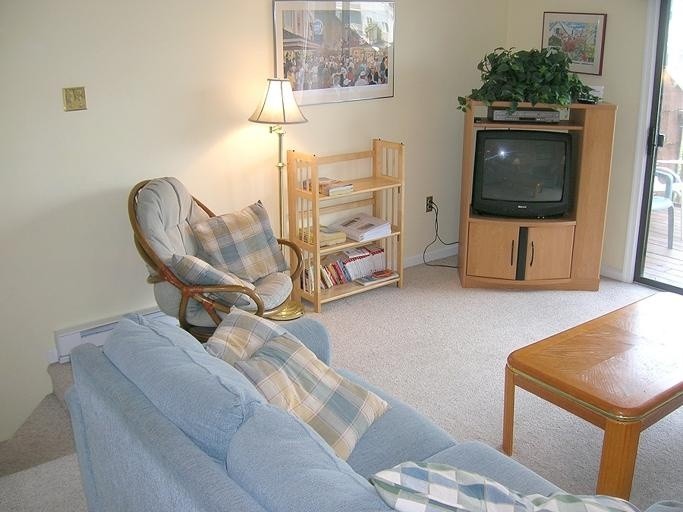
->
<box><xmin>472</xmin><ymin>129</ymin><xmax>572</xmax><ymax>219</ymax></box>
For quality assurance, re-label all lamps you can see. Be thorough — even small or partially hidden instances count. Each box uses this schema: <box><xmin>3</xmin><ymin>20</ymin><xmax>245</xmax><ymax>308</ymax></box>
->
<box><xmin>247</xmin><ymin>78</ymin><xmax>310</xmax><ymax>321</ymax></box>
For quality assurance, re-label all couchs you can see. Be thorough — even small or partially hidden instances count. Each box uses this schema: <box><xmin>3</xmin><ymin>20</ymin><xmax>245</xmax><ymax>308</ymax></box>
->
<box><xmin>63</xmin><ymin>306</ymin><xmax>681</xmax><ymax>512</ymax></box>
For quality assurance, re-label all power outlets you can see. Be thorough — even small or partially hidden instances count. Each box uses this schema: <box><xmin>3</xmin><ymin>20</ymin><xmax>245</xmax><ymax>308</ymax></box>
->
<box><xmin>425</xmin><ymin>195</ymin><xmax>435</xmax><ymax>212</ymax></box>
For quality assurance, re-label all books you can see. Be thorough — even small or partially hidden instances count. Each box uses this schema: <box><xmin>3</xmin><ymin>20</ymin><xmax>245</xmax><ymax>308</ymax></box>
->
<box><xmin>302</xmin><ymin>176</ymin><xmax>353</xmax><ymax>196</ymax></box>
<box><xmin>295</xmin><ymin>210</ymin><xmax>399</xmax><ymax>293</ymax></box>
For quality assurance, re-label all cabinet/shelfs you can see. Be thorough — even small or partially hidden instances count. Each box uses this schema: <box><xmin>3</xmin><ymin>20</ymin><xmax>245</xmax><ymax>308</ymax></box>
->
<box><xmin>456</xmin><ymin>101</ymin><xmax>616</xmax><ymax>290</ymax></box>
<box><xmin>285</xmin><ymin>138</ymin><xmax>405</xmax><ymax>313</ymax></box>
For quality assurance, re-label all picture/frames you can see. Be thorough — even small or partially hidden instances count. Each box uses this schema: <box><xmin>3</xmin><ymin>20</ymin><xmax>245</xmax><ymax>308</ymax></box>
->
<box><xmin>271</xmin><ymin>1</ymin><xmax>395</xmax><ymax>109</ymax></box>
<box><xmin>540</xmin><ymin>11</ymin><xmax>607</xmax><ymax>76</ymax></box>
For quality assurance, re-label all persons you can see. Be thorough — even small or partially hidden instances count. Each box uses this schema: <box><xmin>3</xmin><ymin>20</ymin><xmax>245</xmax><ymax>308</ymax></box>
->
<box><xmin>281</xmin><ymin>36</ymin><xmax>390</xmax><ymax>93</ymax></box>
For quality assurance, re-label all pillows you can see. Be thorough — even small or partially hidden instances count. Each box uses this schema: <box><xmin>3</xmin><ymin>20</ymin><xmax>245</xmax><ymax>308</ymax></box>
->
<box><xmin>369</xmin><ymin>459</ymin><xmax>641</xmax><ymax>512</ymax></box>
<box><xmin>170</xmin><ymin>200</ymin><xmax>290</xmax><ymax>307</ymax></box>
<box><xmin>201</xmin><ymin>303</ymin><xmax>391</xmax><ymax>464</ymax></box>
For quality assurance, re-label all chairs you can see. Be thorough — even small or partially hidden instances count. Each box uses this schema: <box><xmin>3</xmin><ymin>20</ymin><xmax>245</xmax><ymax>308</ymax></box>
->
<box><xmin>648</xmin><ymin>168</ymin><xmax>675</xmax><ymax>250</ymax></box>
<box><xmin>125</xmin><ymin>176</ymin><xmax>304</xmax><ymax>344</ymax></box>
